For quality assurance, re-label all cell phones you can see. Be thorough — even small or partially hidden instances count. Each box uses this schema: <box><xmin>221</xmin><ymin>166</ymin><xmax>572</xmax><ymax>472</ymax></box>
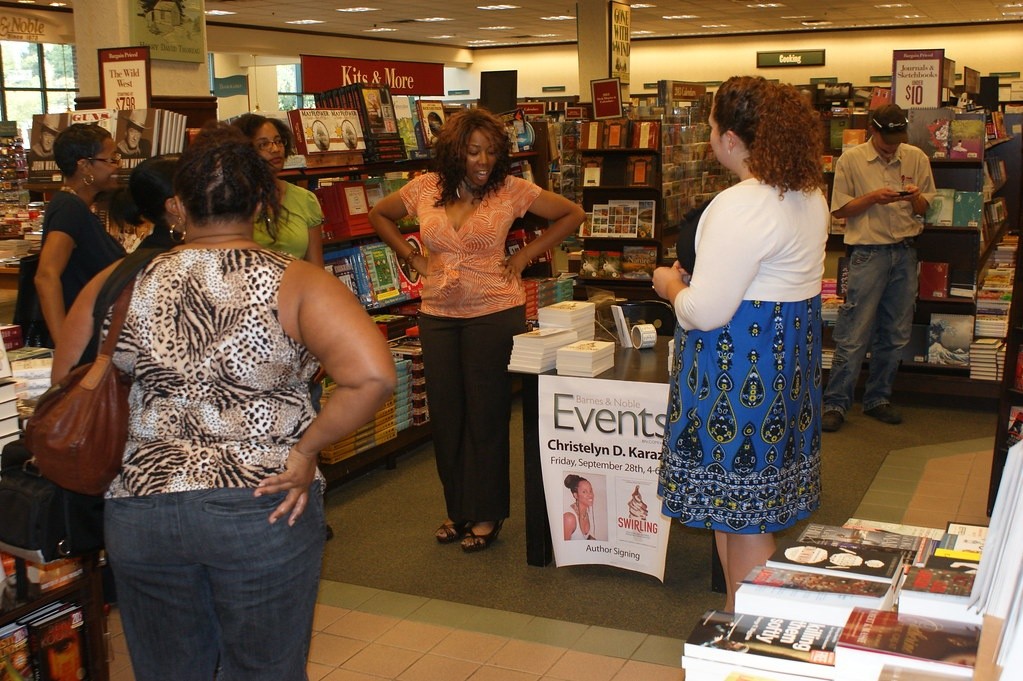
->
<box><xmin>897</xmin><ymin>190</ymin><xmax>912</xmax><ymax>197</ymax></box>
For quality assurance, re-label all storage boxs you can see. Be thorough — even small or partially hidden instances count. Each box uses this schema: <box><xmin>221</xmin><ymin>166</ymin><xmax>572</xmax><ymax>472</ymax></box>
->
<box><xmin>284</xmin><ymin>108</ymin><xmax>419</xmax><ymax>338</ymax></box>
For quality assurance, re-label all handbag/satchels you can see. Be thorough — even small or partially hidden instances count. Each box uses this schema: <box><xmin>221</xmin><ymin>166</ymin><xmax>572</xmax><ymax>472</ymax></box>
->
<box><xmin>13</xmin><ymin>197</ymin><xmax>90</xmax><ymax>349</ymax></box>
<box><xmin>24</xmin><ymin>279</ymin><xmax>135</xmax><ymax>494</ymax></box>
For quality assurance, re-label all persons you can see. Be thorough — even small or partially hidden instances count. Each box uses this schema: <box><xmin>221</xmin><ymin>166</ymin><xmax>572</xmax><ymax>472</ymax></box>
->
<box><xmin>821</xmin><ymin>102</ymin><xmax>937</xmax><ymax>433</ymax></box>
<box><xmin>230</xmin><ymin>114</ymin><xmax>336</xmax><ymax>542</ymax></box>
<box><xmin>99</xmin><ymin>153</ymin><xmax>188</xmax><ymax>247</ymax></box>
<box><xmin>652</xmin><ymin>75</ymin><xmax>831</xmax><ymax>613</ymax></box>
<box><xmin>368</xmin><ymin>111</ymin><xmax>587</xmax><ymax>551</ymax></box>
<box><xmin>31</xmin><ymin>114</ymin><xmax>60</xmax><ymax>160</ymax></box>
<box><xmin>116</xmin><ymin>110</ymin><xmax>151</xmax><ymax>158</ymax></box>
<box><xmin>50</xmin><ymin>121</ymin><xmax>398</xmax><ymax>681</ymax></box>
<box><xmin>563</xmin><ymin>474</ymin><xmax>595</xmax><ymax>541</ymax></box>
<box><xmin>33</xmin><ymin>123</ymin><xmax>127</xmax><ymax>350</ymax></box>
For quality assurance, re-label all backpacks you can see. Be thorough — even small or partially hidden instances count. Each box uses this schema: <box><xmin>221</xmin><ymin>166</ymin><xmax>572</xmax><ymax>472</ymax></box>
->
<box><xmin>0</xmin><ymin>440</ymin><xmax>61</xmax><ymax>562</ymax></box>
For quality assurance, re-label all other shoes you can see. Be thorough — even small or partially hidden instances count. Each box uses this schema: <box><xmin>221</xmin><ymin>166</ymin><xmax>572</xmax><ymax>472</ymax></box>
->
<box><xmin>324</xmin><ymin>525</ymin><xmax>334</xmax><ymax>541</ymax></box>
<box><xmin>864</xmin><ymin>404</ymin><xmax>901</xmax><ymax>423</ymax></box>
<box><xmin>821</xmin><ymin>410</ymin><xmax>844</xmax><ymax>431</ymax></box>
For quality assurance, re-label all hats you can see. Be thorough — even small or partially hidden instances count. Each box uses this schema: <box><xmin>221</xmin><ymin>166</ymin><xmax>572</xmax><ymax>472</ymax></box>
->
<box><xmin>872</xmin><ymin>103</ymin><xmax>909</xmax><ymax>145</ymax></box>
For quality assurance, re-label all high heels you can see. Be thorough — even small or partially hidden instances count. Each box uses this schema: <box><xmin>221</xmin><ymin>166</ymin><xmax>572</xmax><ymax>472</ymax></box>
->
<box><xmin>435</xmin><ymin>519</ymin><xmax>470</xmax><ymax>543</ymax></box>
<box><xmin>460</xmin><ymin>518</ymin><xmax>504</xmax><ymax>550</ymax></box>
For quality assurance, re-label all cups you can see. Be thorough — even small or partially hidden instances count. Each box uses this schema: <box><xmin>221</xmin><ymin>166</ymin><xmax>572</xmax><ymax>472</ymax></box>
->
<box><xmin>631</xmin><ymin>323</ymin><xmax>657</xmax><ymax>349</ymax></box>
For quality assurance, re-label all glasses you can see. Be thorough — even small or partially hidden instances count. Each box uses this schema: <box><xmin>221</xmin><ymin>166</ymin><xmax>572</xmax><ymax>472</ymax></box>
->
<box><xmin>84</xmin><ymin>156</ymin><xmax>123</xmax><ymax>168</ymax></box>
<box><xmin>256</xmin><ymin>137</ymin><xmax>287</xmax><ymax>151</ymax></box>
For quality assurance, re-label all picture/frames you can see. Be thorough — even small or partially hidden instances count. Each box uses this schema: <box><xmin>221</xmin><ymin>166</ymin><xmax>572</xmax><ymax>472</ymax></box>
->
<box><xmin>590</xmin><ymin>76</ymin><xmax>624</xmax><ymax>121</ymax></box>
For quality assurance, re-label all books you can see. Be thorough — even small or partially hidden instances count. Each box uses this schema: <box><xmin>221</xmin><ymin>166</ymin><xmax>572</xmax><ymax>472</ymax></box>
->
<box><xmin>680</xmin><ymin>404</ymin><xmax>1023</xmax><ymax>681</ymax></box>
<box><xmin>0</xmin><ymin>120</ymin><xmax>43</xmax><ymax>267</ymax></box>
<box><xmin>28</xmin><ymin>108</ymin><xmax>188</xmax><ymax>184</ymax></box>
<box><xmin>907</xmin><ymin>107</ymin><xmax>1023</xmax><ymax>381</ymax></box>
<box><xmin>0</xmin><ymin>323</ymin><xmax>100</xmax><ymax>681</ymax></box>
<box><xmin>270</xmin><ymin>83</ymin><xmax>445</xmax><ymax>464</ymax></box>
<box><xmin>508</xmin><ymin>78</ymin><xmax>733</xmax><ymax>376</ymax></box>
<box><xmin>820</xmin><ymin>209</ymin><xmax>850</xmax><ymax>369</ymax></box>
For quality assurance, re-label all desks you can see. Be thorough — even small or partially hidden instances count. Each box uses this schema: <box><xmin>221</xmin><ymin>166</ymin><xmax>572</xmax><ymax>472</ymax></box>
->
<box><xmin>520</xmin><ymin>333</ymin><xmax>675</xmax><ymax>564</ymax></box>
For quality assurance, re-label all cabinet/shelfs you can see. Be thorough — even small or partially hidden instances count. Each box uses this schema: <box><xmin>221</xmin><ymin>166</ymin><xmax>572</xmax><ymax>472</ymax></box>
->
<box><xmin>815</xmin><ymin>155</ymin><xmax>983</xmax><ymax>376</ymax></box>
<box><xmin>579</xmin><ymin>147</ymin><xmax>660</xmax><ymax>289</ymax></box>
<box><xmin>0</xmin><ymin>123</ymin><xmax>552</xmax><ymax>628</ymax></box>
